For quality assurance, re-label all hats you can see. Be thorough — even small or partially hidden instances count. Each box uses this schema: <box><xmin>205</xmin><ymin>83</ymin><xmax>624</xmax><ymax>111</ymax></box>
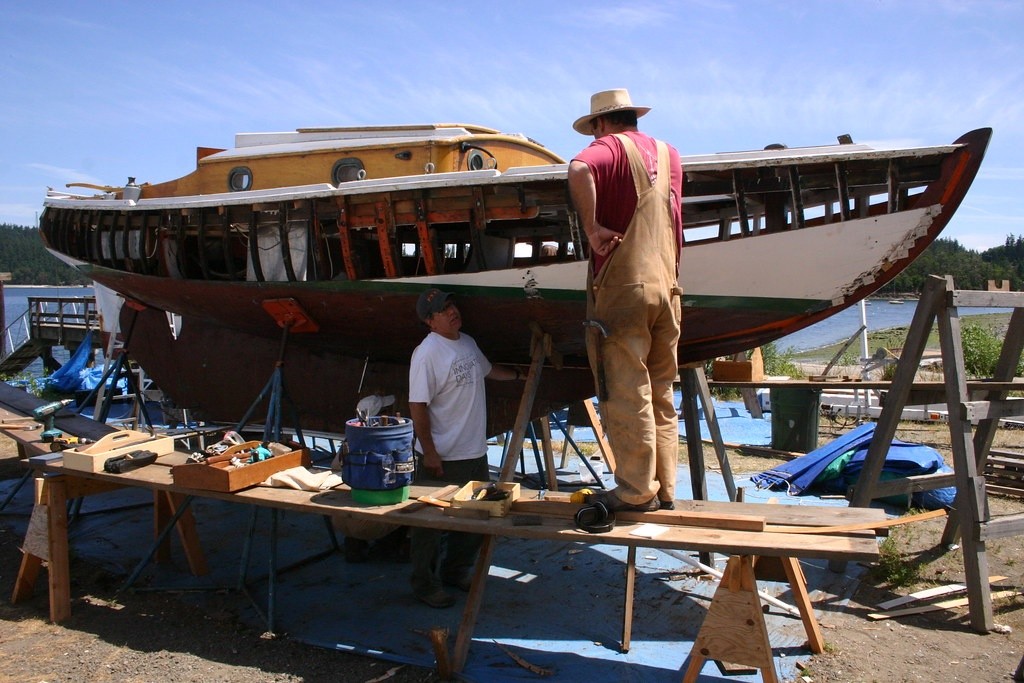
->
<box><xmin>416</xmin><ymin>288</ymin><xmax>456</xmax><ymax>321</ymax></box>
<box><xmin>573</xmin><ymin>89</ymin><xmax>653</xmax><ymax>136</ymax></box>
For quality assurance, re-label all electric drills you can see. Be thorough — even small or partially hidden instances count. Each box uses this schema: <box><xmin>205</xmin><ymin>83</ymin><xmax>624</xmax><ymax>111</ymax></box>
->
<box><xmin>32</xmin><ymin>400</ymin><xmax>97</xmax><ymax>453</ymax></box>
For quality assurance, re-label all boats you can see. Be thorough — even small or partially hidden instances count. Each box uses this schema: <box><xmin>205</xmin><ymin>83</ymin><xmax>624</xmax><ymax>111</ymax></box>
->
<box><xmin>36</xmin><ymin>121</ymin><xmax>993</xmax><ymax>441</ymax></box>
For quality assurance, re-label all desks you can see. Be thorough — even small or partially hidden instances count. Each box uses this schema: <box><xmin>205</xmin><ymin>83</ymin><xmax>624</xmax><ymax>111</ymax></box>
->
<box><xmin>0</xmin><ymin>378</ymin><xmax>893</xmax><ymax>683</ymax></box>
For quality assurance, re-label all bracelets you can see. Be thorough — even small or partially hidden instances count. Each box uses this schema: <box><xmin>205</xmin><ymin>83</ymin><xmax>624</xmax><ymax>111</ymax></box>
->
<box><xmin>513</xmin><ymin>369</ymin><xmax>521</xmax><ymax>381</ymax></box>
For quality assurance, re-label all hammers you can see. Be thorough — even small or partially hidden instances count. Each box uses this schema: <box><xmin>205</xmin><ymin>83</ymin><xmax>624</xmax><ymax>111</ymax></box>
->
<box><xmin>583</xmin><ymin>319</ymin><xmax>611</xmax><ymax>402</ymax></box>
<box><xmin>475</xmin><ymin>482</ymin><xmax>498</xmax><ymax>501</ymax></box>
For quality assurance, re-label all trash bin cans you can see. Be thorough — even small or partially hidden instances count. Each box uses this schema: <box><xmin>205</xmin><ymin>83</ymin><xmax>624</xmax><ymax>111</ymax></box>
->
<box><xmin>769</xmin><ymin>387</ymin><xmax>823</xmax><ymax>455</ymax></box>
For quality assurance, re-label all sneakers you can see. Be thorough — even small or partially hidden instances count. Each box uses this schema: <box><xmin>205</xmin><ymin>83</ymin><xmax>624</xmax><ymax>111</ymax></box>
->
<box><xmin>440</xmin><ymin>568</ymin><xmax>472</xmax><ymax>594</ymax></box>
<box><xmin>583</xmin><ymin>489</ymin><xmax>676</xmax><ymax>512</ymax></box>
<box><xmin>414</xmin><ymin>582</ymin><xmax>457</xmax><ymax>609</ymax></box>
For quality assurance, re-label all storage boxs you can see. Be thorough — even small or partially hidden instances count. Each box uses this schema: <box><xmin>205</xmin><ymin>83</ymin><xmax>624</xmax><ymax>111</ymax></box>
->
<box><xmin>450</xmin><ymin>481</ymin><xmax>521</xmax><ymax>517</ymax></box>
<box><xmin>173</xmin><ymin>440</ymin><xmax>311</xmax><ymax>494</ymax></box>
<box><xmin>62</xmin><ymin>431</ymin><xmax>174</xmax><ymax>472</ymax></box>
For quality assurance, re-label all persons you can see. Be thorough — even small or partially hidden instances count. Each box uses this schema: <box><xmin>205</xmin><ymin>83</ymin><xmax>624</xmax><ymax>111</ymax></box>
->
<box><xmin>406</xmin><ymin>286</ymin><xmax>531</xmax><ymax>609</ymax></box>
<box><xmin>569</xmin><ymin>87</ymin><xmax>686</xmax><ymax>514</ymax></box>
